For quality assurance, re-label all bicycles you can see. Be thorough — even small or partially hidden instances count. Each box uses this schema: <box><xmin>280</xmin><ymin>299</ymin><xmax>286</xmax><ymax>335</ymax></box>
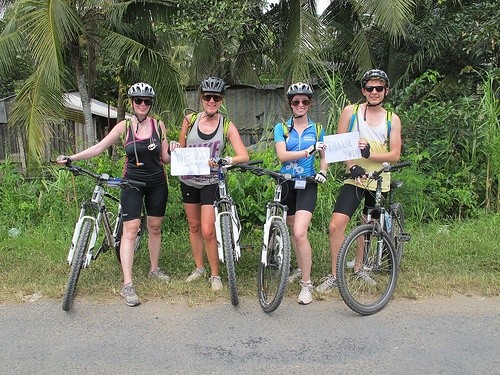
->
<box><xmin>335</xmin><ymin>162</ymin><xmax>414</xmax><ymax>315</ymax></box>
<box><xmin>210</xmin><ymin>157</ymin><xmax>264</xmax><ymax>308</ymax></box>
<box><xmin>236</xmin><ymin>162</ymin><xmax>320</xmax><ymax>312</ymax></box>
<box><xmin>49</xmin><ymin>157</ymin><xmax>149</xmax><ymax>311</ymax></box>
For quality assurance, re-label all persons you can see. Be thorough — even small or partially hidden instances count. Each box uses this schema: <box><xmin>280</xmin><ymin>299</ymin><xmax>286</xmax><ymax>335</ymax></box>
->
<box><xmin>316</xmin><ymin>69</ymin><xmax>402</xmax><ymax>294</ymax></box>
<box><xmin>274</xmin><ymin>82</ymin><xmax>328</xmax><ymax>305</ymax></box>
<box><xmin>56</xmin><ymin>82</ymin><xmax>181</xmax><ymax>307</ymax></box>
<box><xmin>180</xmin><ymin>76</ymin><xmax>250</xmax><ymax>290</ymax></box>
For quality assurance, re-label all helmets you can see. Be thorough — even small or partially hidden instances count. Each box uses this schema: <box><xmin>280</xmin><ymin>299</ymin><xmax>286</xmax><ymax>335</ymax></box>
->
<box><xmin>128</xmin><ymin>83</ymin><xmax>155</xmax><ymax>97</ymax></box>
<box><xmin>360</xmin><ymin>69</ymin><xmax>390</xmax><ymax>88</ymax></box>
<box><xmin>200</xmin><ymin>76</ymin><xmax>226</xmax><ymax>93</ymax></box>
<box><xmin>286</xmin><ymin>82</ymin><xmax>313</xmax><ymax>97</ymax></box>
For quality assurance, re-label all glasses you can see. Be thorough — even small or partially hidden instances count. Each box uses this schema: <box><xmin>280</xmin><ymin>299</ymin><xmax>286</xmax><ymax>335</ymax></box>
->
<box><xmin>133</xmin><ymin>98</ymin><xmax>152</xmax><ymax>106</ymax></box>
<box><xmin>291</xmin><ymin>100</ymin><xmax>310</xmax><ymax>106</ymax></box>
<box><xmin>364</xmin><ymin>85</ymin><xmax>386</xmax><ymax>92</ymax></box>
<box><xmin>201</xmin><ymin>94</ymin><xmax>223</xmax><ymax>102</ymax></box>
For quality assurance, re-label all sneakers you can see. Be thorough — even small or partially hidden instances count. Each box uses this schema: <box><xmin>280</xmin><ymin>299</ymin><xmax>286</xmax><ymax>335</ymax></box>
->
<box><xmin>287</xmin><ymin>267</ymin><xmax>303</xmax><ymax>283</ymax></box>
<box><xmin>186</xmin><ymin>267</ymin><xmax>206</xmax><ymax>282</ymax></box>
<box><xmin>351</xmin><ymin>267</ymin><xmax>378</xmax><ymax>286</ymax></box>
<box><xmin>297</xmin><ymin>280</ymin><xmax>313</xmax><ymax>304</ymax></box>
<box><xmin>315</xmin><ymin>273</ymin><xmax>339</xmax><ymax>293</ymax></box>
<box><xmin>208</xmin><ymin>275</ymin><xmax>223</xmax><ymax>291</ymax></box>
<box><xmin>148</xmin><ymin>267</ymin><xmax>169</xmax><ymax>281</ymax></box>
<box><xmin>120</xmin><ymin>282</ymin><xmax>141</xmax><ymax>307</ymax></box>
